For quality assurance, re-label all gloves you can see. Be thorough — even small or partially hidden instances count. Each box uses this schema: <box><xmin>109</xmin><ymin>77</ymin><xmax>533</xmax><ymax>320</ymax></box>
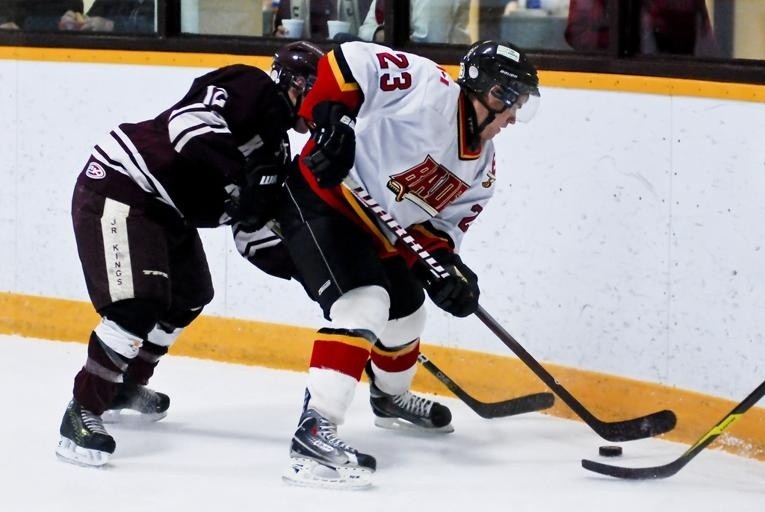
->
<box><xmin>424</xmin><ymin>252</ymin><xmax>480</xmax><ymax>318</ymax></box>
<box><xmin>304</xmin><ymin>100</ymin><xmax>359</xmax><ymax>189</ymax></box>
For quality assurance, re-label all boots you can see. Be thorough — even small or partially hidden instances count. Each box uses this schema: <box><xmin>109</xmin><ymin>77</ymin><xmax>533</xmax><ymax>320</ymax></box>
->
<box><xmin>364</xmin><ymin>357</ymin><xmax>452</xmax><ymax>429</ymax></box>
<box><xmin>288</xmin><ymin>385</ymin><xmax>377</xmax><ymax>472</ymax></box>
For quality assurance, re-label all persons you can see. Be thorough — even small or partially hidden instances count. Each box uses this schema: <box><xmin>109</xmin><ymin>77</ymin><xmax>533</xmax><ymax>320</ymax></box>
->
<box><xmin>20</xmin><ymin>0</ymin><xmax>166</xmax><ymax>37</ymax></box>
<box><xmin>60</xmin><ymin>41</ymin><xmax>326</xmax><ymax>454</ymax></box>
<box><xmin>357</xmin><ymin>0</ymin><xmax>472</xmax><ymax>44</ymax></box>
<box><xmin>231</xmin><ymin>38</ymin><xmax>540</xmax><ymax>471</ymax></box>
<box><xmin>267</xmin><ymin>0</ymin><xmax>365</xmax><ymax>41</ymax></box>
<box><xmin>563</xmin><ymin>1</ymin><xmax>721</xmax><ymax>59</ymax></box>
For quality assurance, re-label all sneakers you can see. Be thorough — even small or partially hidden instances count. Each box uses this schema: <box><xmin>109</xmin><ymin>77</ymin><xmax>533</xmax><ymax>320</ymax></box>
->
<box><xmin>109</xmin><ymin>383</ymin><xmax>171</xmax><ymax>413</ymax></box>
<box><xmin>58</xmin><ymin>398</ymin><xmax>118</xmax><ymax>455</ymax></box>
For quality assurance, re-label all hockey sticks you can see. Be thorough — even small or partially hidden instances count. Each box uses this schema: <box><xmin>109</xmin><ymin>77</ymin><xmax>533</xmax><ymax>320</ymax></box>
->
<box><xmin>222</xmin><ymin>197</ymin><xmax>554</xmax><ymax>419</ymax></box>
<box><xmin>311</xmin><ymin>143</ymin><xmax>677</xmax><ymax>441</ymax></box>
<box><xmin>582</xmin><ymin>381</ymin><xmax>765</xmax><ymax>479</ymax></box>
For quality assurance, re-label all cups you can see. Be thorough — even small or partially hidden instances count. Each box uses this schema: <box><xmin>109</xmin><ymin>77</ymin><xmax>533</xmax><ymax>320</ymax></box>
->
<box><xmin>283</xmin><ymin>19</ymin><xmax>304</xmax><ymax>38</ymax></box>
<box><xmin>326</xmin><ymin>21</ymin><xmax>351</xmax><ymax>39</ymax></box>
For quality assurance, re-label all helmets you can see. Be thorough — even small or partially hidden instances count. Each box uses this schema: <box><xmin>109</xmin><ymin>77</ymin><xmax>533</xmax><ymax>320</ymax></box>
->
<box><xmin>455</xmin><ymin>38</ymin><xmax>541</xmax><ymax>114</ymax></box>
<box><xmin>269</xmin><ymin>40</ymin><xmax>328</xmax><ymax>110</ymax></box>
